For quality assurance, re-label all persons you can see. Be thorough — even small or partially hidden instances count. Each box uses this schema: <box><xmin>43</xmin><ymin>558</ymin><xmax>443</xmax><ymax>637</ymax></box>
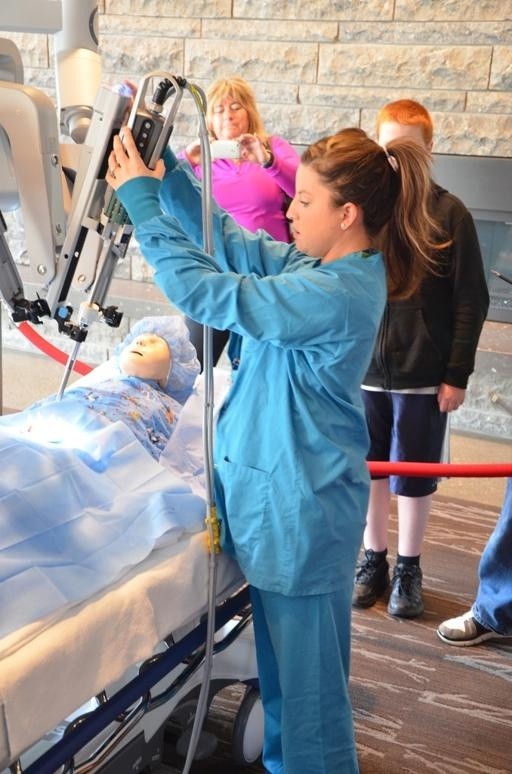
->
<box><xmin>437</xmin><ymin>477</ymin><xmax>512</xmax><ymax>646</ymax></box>
<box><xmin>352</xmin><ymin>99</ymin><xmax>490</xmax><ymax>620</ymax></box>
<box><xmin>177</xmin><ymin>76</ymin><xmax>300</xmax><ymax>373</ymax></box>
<box><xmin>106</xmin><ymin>126</ymin><xmax>452</xmax><ymax>774</ymax></box>
<box><xmin>26</xmin><ymin>333</ymin><xmax>183</xmax><ymax>461</ymax></box>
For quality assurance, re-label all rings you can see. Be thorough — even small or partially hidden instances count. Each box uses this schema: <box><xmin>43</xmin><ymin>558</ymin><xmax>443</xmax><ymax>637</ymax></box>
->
<box><xmin>110</xmin><ymin>163</ymin><xmax>119</xmax><ymax>177</ymax></box>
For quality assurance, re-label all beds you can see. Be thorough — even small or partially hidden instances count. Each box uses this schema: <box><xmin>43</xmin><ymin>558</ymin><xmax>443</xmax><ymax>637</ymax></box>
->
<box><xmin>1</xmin><ymin>349</ymin><xmax>265</xmax><ymax>774</ymax></box>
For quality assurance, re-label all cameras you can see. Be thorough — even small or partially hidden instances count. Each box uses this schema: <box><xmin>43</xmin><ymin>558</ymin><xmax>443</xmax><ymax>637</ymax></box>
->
<box><xmin>209</xmin><ymin>138</ymin><xmax>241</xmax><ymax>158</ymax></box>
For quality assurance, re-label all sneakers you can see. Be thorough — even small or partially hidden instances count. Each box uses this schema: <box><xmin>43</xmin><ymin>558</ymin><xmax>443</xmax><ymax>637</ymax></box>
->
<box><xmin>437</xmin><ymin>609</ymin><xmax>512</xmax><ymax>647</ymax></box>
<box><xmin>351</xmin><ymin>549</ymin><xmax>425</xmax><ymax>618</ymax></box>
